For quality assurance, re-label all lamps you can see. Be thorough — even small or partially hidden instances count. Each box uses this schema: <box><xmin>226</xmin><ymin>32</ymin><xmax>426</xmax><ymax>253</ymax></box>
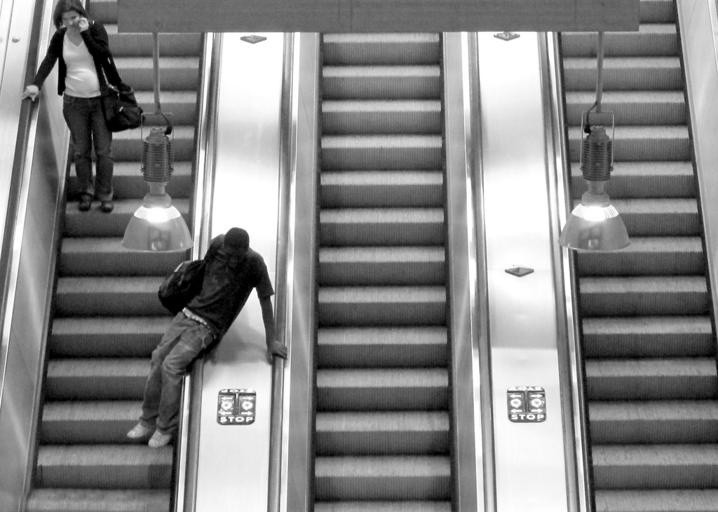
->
<box><xmin>556</xmin><ymin>31</ymin><xmax>633</xmax><ymax>254</ymax></box>
<box><xmin>119</xmin><ymin>29</ymin><xmax>196</xmax><ymax>256</ymax></box>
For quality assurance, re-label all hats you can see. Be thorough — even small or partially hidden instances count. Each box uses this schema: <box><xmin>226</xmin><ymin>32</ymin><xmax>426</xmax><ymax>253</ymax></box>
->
<box><xmin>224</xmin><ymin>228</ymin><xmax>249</xmax><ymax>255</ymax></box>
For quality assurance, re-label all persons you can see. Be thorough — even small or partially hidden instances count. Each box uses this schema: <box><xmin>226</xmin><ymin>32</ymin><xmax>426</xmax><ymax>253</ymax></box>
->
<box><xmin>124</xmin><ymin>228</ymin><xmax>287</xmax><ymax>448</ymax></box>
<box><xmin>20</xmin><ymin>0</ymin><xmax>116</xmax><ymax>212</ymax></box>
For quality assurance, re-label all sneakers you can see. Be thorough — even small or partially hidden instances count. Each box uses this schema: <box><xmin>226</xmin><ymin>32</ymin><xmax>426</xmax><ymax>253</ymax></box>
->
<box><xmin>147</xmin><ymin>428</ymin><xmax>174</xmax><ymax>448</ymax></box>
<box><xmin>126</xmin><ymin>420</ymin><xmax>156</xmax><ymax>440</ymax></box>
<box><xmin>100</xmin><ymin>198</ymin><xmax>113</xmax><ymax>213</ymax></box>
<box><xmin>77</xmin><ymin>190</ymin><xmax>93</xmax><ymax>211</ymax></box>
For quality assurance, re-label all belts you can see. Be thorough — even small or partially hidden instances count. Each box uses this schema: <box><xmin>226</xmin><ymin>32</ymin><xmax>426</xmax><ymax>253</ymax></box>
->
<box><xmin>180</xmin><ymin>308</ymin><xmax>218</xmax><ymax>340</ymax></box>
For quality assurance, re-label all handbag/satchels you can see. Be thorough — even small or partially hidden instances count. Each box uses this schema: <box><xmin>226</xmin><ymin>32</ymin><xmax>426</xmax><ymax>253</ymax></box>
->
<box><xmin>100</xmin><ymin>80</ymin><xmax>146</xmax><ymax>132</ymax></box>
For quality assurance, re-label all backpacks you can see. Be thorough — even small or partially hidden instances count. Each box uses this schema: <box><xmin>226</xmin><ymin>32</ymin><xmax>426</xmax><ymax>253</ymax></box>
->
<box><xmin>157</xmin><ymin>260</ymin><xmax>206</xmax><ymax>315</ymax></box>
